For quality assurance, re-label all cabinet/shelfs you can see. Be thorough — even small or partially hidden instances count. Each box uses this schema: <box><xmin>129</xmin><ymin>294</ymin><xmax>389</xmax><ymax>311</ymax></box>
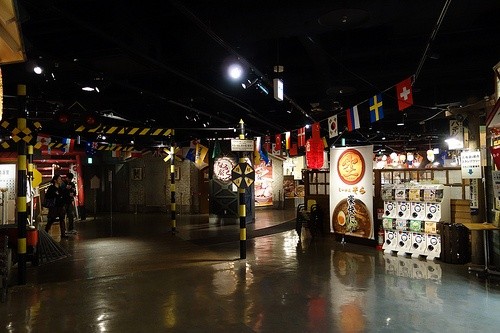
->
<box><xmin>301</xmin><ymin>168</ymin><xmax>473</xmax><ymax>264</ymax></box>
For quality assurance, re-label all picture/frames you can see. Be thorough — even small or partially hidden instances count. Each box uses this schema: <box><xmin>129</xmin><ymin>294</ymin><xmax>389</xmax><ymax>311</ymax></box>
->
<box><xmin>132</xmin><ymin>168</ymin><xmax>142</xmax><ymax>181</ymax></box>
<box><xmin>167</xmin><ymin>166</ymin><xmax>180</xmax><ymax>180</ymax></box>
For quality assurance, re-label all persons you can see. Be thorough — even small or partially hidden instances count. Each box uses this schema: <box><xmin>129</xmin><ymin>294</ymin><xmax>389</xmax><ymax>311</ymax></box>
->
<box><xmin>45</xmin><ymin>175</ymin><xmax>69</xmax><ymax>238</ymax></box>
<box><xmin>61</xmin><ymin>173</ymin><xmax>78</xmax><ymax>234</ymax></box>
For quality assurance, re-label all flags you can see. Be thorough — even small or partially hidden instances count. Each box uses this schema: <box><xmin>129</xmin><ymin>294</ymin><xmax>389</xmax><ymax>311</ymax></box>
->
<box><xmin>264</xmin><ymin>77</ymin><xmax>412</xmax><ymax>151</ymax></box>
<box><xmin>35</xmin><ymin>135</ymin><xmax>208</xmax><ymax>164</ymax></box>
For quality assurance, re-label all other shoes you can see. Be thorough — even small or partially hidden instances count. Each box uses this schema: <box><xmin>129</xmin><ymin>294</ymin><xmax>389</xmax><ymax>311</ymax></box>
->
<box><xmin>61</xmin><ymin>235</ymin><xmax>69</xmax><ymax>239</ymax></box>
<box><xmin>64</xmin><ymin>229</ymin><xmax>78</xmax><ymax>234</ymax></box>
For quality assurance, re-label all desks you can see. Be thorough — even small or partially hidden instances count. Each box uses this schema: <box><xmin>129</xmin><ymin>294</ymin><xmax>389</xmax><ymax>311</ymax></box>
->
<box><xmin>464</xmin><ymin>222</ymin><xmax>500</xmax><ymax>277</ymax></box>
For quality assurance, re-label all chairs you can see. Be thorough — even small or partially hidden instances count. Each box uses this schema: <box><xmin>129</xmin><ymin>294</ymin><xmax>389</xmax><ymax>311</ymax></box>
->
<box><xmin>296</xmin><ymin>199</ymin><xmax>321</xmax><ymax>243</ymax></box>
